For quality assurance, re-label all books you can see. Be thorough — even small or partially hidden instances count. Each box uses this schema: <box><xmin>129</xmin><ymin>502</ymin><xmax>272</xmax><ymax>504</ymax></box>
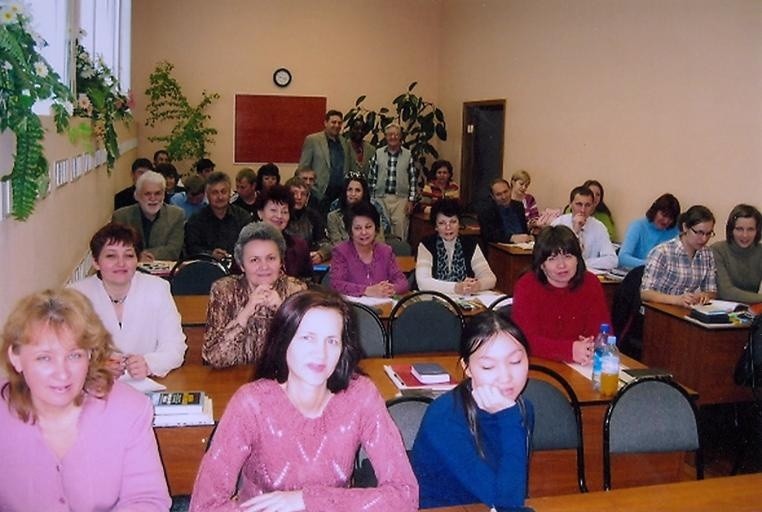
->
<box><xmin>143</xmin><ymin>389</ymin><xmax>216</xmax><ymax>427</ymax></box>
<box><xmin>684</xmin><ymin>305</ymin><xmax>734</xmax><ymax>329</ymax></box>
<box><xmin>383</xmin><ymin>359</ymin><xmax>459</xmax><ymax>391</ymax></box>
<box><xmin>707</xmin><ymin>299</ymin><xmax>749</xmax><ymax>312</ymax></box>
<box><xmin>617</xmin><ymin>368</ymin><xmax>673</xmax><ymax>385</ymax></box>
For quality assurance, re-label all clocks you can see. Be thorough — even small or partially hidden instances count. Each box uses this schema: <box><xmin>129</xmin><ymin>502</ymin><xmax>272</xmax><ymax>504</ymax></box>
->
<box><xmin>273</xmin><ymin>68</ymin><xmax>293</xmax><ymax>88</ymax></box>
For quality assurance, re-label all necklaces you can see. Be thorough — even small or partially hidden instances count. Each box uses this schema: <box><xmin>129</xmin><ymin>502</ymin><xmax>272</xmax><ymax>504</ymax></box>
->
<box><xmin>110</xmin><ymin>296</ymin><xmax>125</xmax><ymax>303</ymax></box>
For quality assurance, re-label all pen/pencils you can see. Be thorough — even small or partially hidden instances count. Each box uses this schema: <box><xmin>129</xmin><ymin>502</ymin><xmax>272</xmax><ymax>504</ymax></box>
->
<box><xmin>468</xmin><ymin>278</ymin><xmax>479</xmax><ymax>284</ymax></box>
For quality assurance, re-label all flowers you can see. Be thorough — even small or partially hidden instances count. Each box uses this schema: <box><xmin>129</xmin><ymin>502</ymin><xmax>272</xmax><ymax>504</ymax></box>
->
<box><xmin>70</xmin><ymin>25</ymin><xmax>137</xmax><ymax>141</ymax></box>
<box><xmin>0</xmin><ymin>1</ymin><xmax>55</xmax><ymax>80</ymax></box>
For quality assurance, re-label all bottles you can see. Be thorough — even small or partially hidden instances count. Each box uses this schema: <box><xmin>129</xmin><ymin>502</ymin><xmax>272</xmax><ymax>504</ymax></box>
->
<box><xmin>599</xmin><ymin>334</ymin><xmax>621</xmax><ymax>397</ymax></box>
<box><xmin>590</xmin><ymin>323</ymin><xmax>610</xmax><ymax>391</ymax></box>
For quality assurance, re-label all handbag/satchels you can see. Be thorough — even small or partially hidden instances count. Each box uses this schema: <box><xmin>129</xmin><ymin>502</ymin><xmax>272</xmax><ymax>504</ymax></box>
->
<box><xmin>537</xmin><ymin>208</ymin><xmax>561</xmax><ymax>225</ymax></box>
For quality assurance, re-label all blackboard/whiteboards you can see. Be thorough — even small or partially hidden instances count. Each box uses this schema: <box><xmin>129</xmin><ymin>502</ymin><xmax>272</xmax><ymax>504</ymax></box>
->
<box><xmin>233</xmin><ymin>93</ymin><xmax>328</xmax><ymax>164</ymax></box>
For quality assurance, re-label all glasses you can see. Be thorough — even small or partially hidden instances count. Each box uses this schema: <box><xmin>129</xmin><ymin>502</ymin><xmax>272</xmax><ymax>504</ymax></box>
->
<box><xmin>691</xmin><ymin>228</ymin><xmax>715</xmax><ymax>238</ymax></box>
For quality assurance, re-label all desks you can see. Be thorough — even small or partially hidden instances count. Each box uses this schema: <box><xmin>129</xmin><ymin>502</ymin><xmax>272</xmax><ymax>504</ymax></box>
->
<box><xmin>133</xmin><ymin>206</ymin><xmax>762</xmax><ymax>512</ymax></box>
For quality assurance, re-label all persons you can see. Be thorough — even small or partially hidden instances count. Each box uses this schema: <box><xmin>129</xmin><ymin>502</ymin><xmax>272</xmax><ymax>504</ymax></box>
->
<box><xmin>404</xmin><ymin>312</ymin><xmax>533</xmax><ymax>511</ymax></box>
<box><xmin>200</xmin><ymin>226</ymin><xmax>308</xmax><ymax>368</ymax></box>
<box><xmin>616</xmin><ymin>192</ymin><xmax>761</xmax><ymax>305</ymax></box>
<box><xmin>56</xmin><ymin>223</ymin><xmax>187</xmax><ymax>380</ymax></box>
<box><xmin>487</xmin><ymin>170</ymin><xmax>541</xmax><ymax>245</ymax></box>
<box><xmin>298</xmin><ymin>110</ymin><xmax>416</xmax><ymax>243</ymax></box>
<box><xmin>552</xmin><ymin>180</ymin><xmax>621</xmax><ymax>270</ymax></box>
<box><xmin>421</xmin><ymin>161</ymin><xmax>462</xmax><ymax>200</ymax></box>
<box><xmin>112</xmin><ymin>148</ymin><xmax>326</xmax><ymax>263</ymax></box>
<box><xmin>327</xmin><ymin>204</ymin><xmax>409</xmax><ymax>296</ymax></box>
<box><xmin>414</xmin><ymin>199</ymin><xmax>498</xmax><ymax>292</ymax></box>
<box><xmin>188</xmin><ymin>289</ymin><xmax>420</xmax><ymax>511</ymax></box>
<box><xmin>508</xmin><ymin>224</ymin><xmax>614</xmax><ymax>367</ymax></box>
<box><xmin>0</xmin><ymin>288</ymin><xmax>171</xmax><ymax>511</ymax></box>
